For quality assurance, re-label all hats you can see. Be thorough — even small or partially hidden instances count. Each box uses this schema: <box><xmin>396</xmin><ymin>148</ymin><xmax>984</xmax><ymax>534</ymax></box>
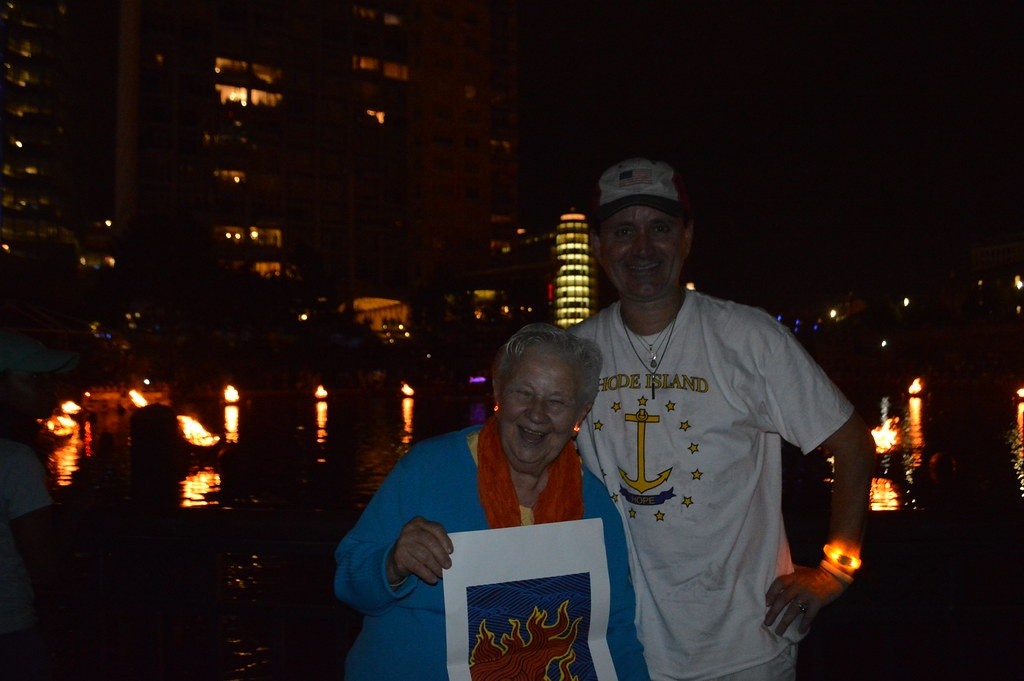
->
<box><xmin>589</xmin><ymin>158</ymin><xmax>689</xmax><ymax>226</ymax></box>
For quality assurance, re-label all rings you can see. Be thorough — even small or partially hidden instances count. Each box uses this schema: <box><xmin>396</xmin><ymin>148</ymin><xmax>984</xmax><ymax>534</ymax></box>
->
<box><xmin>797</xmin><ymin>600</ymin><xmax>808</xmax><ymax>612</ymax></box>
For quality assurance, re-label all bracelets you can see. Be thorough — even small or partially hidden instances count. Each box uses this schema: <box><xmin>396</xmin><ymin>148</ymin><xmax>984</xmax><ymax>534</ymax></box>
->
<box><xmin>820</xmin><ymin>561</ymin><xmax>854</xmax><ymax>584</ymax></box>
<box><xmin>823</xmin><ymin>544</ymin><xmax>861</xmax><ymax>571</ymax></box>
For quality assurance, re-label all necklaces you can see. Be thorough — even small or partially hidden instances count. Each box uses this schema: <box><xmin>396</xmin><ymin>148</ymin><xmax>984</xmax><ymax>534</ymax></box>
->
<box><xmin>620</xmin><ymin>277</ymin><xmax>685</xmax><ymax>399</ymax></box>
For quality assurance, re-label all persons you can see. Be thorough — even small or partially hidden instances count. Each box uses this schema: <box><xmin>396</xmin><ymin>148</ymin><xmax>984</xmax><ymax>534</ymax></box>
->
<box><xmin>564</xmin><ymin>159</ymin><xmax>876</xmax><ymax>681</ymax></box>
<box><xmin>330</xmin><ymin>322</ymin><xmax>651</xmax><ymax>681</ymax></box>
<box><xmin>0</xmin><ymin>331</ymin><xmax>93</xmax><ymax>681</ymax></box>
